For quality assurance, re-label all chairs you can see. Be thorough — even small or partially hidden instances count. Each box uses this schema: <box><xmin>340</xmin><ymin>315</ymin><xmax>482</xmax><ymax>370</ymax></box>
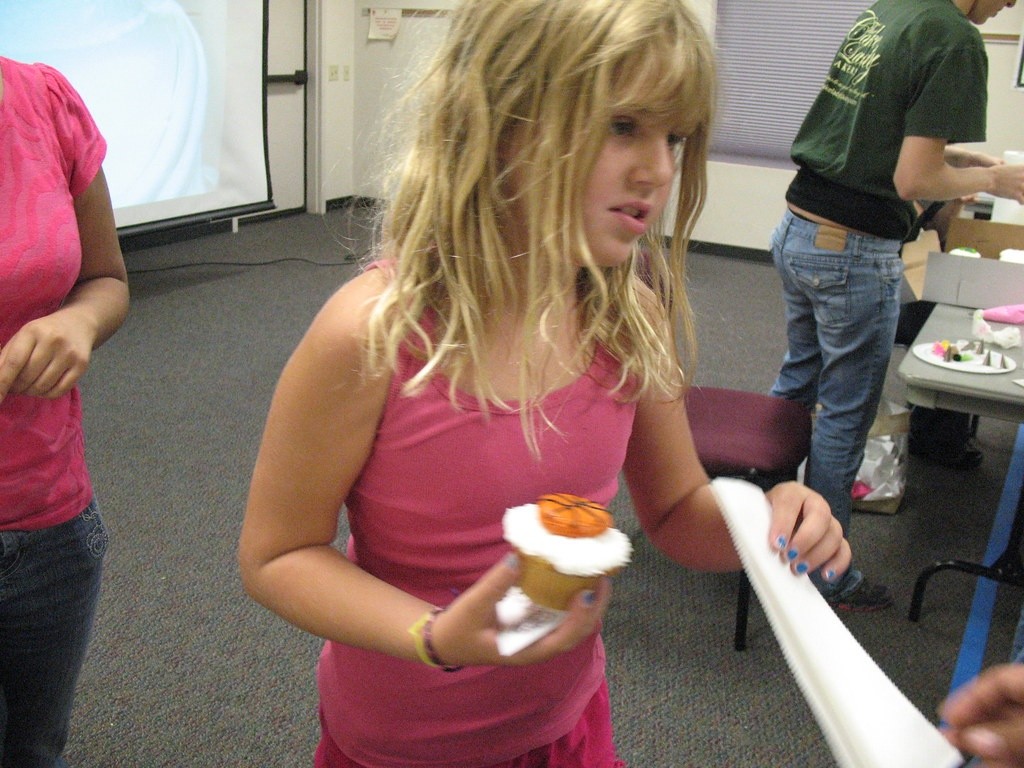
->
<box><xmin>684</xmin><ymin>385</ymin><xmax>813</xmax><ymax>652</ymax></box>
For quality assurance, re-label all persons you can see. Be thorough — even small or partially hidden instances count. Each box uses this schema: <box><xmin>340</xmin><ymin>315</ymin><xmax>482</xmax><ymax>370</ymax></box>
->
<box><xmin>767</xmin><ymin>0</ymin><xmax>1024</xmax><ymax>611</ymax></box>
<box><xmin>0</xmin><ymin>55</ymin><xmax>130</xmax><ymax>768</ymax></box>
<box><xmin>935</xmin><ymin>662</ymin><xmax>1024</xmax><ymax>768</ymax></box>
<box><xmin>236</xmin><ymin>0</ymin><xmax>854</xmax><ymax>768</ymax></box>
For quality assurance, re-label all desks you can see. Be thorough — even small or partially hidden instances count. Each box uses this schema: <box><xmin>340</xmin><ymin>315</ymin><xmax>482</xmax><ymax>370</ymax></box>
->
<box><xmin>899</xmin><ymin>302</ymin><xmax>1024</xmax><ymax>624</ymax></box>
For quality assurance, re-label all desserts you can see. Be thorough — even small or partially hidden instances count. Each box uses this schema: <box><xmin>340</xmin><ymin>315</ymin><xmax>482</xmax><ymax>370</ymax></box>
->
<box><xmin>501</xmin><ymin>493</ymin><xmax>633</xmax><ymax>610</ymax></box>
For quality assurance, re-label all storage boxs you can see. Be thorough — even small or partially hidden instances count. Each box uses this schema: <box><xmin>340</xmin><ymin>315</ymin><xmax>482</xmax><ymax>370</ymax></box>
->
<box><xmin>901</xmin><ymin>218</ymin><xmax>1024</xmax><ymax>308</ymax></box>
<box><xmin>852</xmin><ymin>398</ymin><xmax>910</xmax><ymax>515</ymax></box>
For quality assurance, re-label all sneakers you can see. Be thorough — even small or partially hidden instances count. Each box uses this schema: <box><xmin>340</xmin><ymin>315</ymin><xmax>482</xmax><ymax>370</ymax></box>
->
<box><xmin>820</xmin><ymin>570</ymin><xmax>894</xmax><ymax>613</ymax></box>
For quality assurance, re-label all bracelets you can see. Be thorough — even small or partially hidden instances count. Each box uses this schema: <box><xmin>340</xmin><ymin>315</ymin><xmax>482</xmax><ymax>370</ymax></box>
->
<box><xmin>408</xmin><ymin>611</ymin><xmax>464</xmax><ymax>672</ymax></box>
